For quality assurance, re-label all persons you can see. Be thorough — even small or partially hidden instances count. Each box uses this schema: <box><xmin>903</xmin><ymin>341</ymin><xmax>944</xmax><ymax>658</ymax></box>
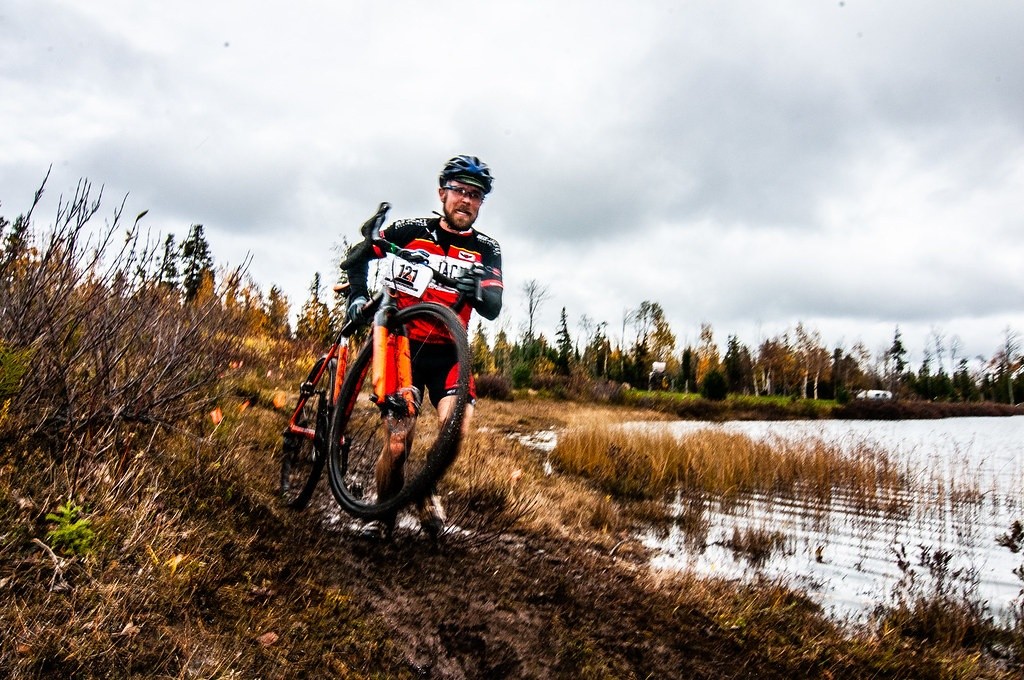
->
<box><xmin>348</xmin><ymin>154</ymin><xmax>503</xmax><ymax>536</ymax></box>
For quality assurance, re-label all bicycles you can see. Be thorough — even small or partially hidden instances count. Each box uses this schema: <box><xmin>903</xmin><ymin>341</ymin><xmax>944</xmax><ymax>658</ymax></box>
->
<box><xmin>276</xmin><ymin>200</ymin><xmax>486</xmax><ymax>522</ymax></box>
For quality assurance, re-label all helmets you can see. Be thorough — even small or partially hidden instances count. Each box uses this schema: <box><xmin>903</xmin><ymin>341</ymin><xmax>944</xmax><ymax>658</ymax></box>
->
<box><xmin>441</xmin><ymin>154</ymin><xmax>492</xmax><ymax>194</ymax></box>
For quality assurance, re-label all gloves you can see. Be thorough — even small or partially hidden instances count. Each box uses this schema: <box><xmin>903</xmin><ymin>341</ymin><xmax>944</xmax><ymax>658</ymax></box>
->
<box><xmin>456</xmin><ymin>272</ymin><xmax>483</xmax><ymax>304</ymax></box>
<box><xmin>346</xmin><ymin>296</ymin><xmax>374</xmax><ymax>327</ymax></box>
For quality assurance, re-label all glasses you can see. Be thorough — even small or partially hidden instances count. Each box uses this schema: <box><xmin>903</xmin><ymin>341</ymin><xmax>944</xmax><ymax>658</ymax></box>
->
<box><xmin>442</xmin><ymin>186</ymin><xmax>485</xmax><ymax>202</ymax></box>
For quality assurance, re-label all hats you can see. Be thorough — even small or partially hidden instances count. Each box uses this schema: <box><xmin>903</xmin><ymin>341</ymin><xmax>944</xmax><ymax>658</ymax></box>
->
<box><xmin>444</xmin><ymin>175</ymin><xmax>486</xmax><ymax>191</ymax></box>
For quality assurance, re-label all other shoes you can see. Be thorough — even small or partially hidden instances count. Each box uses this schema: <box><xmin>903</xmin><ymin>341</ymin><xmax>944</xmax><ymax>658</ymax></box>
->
<box><xmin>358</xmin><ymin>518</ymin><xmax>396</xmax><ymax>546</ymax></box>
<box><xmin>417</xmin><ymin>493</ymin><xmax>446</xmax><ymax>534</ymax></box>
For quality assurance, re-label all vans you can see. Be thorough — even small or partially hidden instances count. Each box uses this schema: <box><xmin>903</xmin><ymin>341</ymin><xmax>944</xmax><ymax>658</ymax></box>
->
<box><xmin>856</xmin><ymin>389</ymin><xmax>892</xmax><ymax>401</ymax></box>
